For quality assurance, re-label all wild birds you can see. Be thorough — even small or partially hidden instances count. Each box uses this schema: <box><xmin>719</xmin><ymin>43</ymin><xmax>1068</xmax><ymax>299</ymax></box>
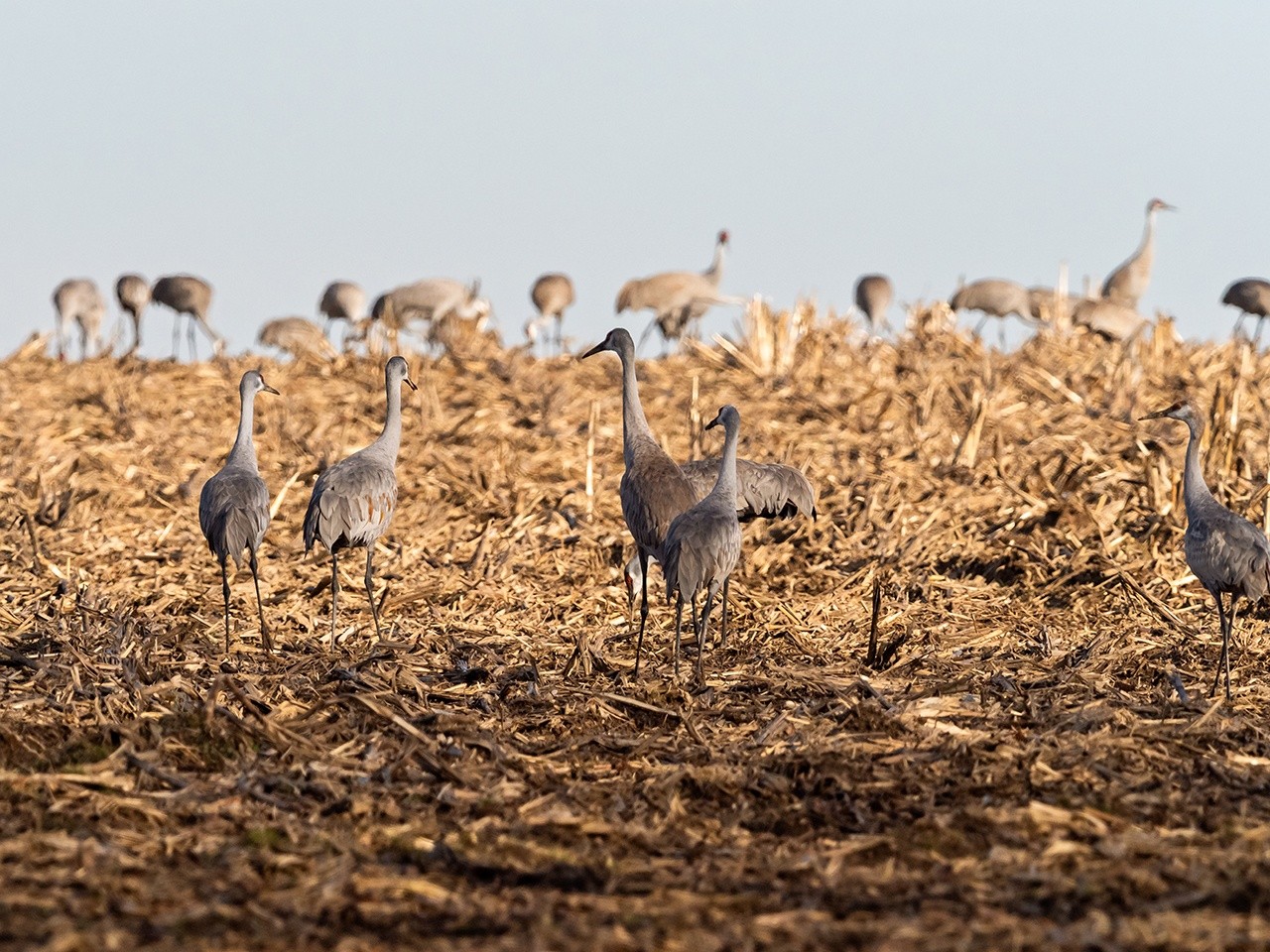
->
<box><xmin>301</xmin><ymin>357</ymin><xmax>421</xmax><ymax>657</ymax></box>
<box><xmin>582</xmin><ymin>327</ymin><xmax>820</xmax><ymax>680</ymax></box>
<box><xmin>200</xmin><ymin>370</ymin><xmax>283</xmax><ymax>655</ymax></box>
<box><xmin>260</xmin><ymin>198</ymin><xmax>1270</xmax><ymax>367</ymax></box>
<box><xmin>1138</xmin><ymin>400</ymin><xmax>1269</xmax><ymax>704</ymax></box>
<box><xmin>53</xmin><ymin>274</ymin><xmax>227</xmax><ymax>362</ymax></box>
